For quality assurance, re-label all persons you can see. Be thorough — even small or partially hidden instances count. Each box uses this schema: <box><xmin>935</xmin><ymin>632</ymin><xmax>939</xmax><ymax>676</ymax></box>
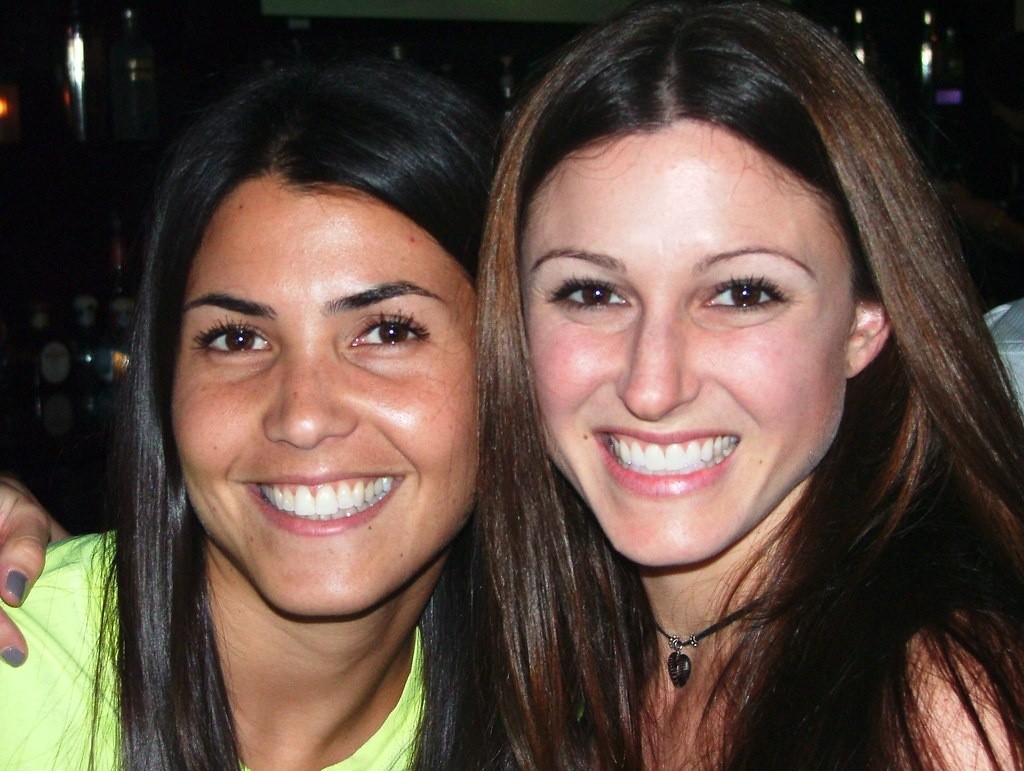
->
<box><xmin>0</xmin><ymin>0</ymin><xmax>1024</xmax><ymax>771</ymax></box>
<box><xmin>939</xmin><ymin>30</ymin><xmax>1024</xmax><ymax>311</ymax></box>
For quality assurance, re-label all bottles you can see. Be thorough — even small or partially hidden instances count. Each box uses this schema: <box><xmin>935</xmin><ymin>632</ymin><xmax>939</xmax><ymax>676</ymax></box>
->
<box><xmin>827</xmin><ymin>6</ymin><xmax>959</xmax><ymax>108</ymax></box>
<box><xmin>172</xmin><ymin>16</ymin><xmax>516</xmax><ymax>138</ymax></box>
<box><xmin>0</xmin><ymin>1</ymin><xmax>160</xmax><ymax>533</ymax></box>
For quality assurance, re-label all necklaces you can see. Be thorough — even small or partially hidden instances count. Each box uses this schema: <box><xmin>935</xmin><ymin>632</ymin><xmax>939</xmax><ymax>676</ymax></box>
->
<box><xmin>655</xmin><ymin>592</ymin><xmax>772</xmax><ymax>689</ymax></box>
